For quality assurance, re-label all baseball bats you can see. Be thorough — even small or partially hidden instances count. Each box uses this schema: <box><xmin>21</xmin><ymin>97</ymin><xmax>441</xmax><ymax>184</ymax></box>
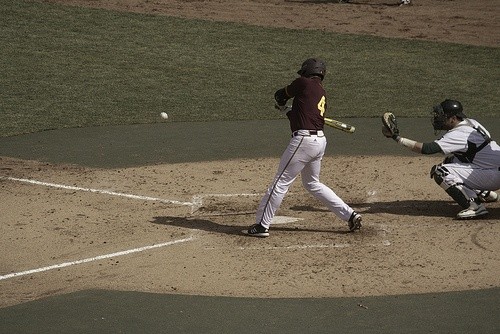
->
<box><xmin>275</xmin><ymin>104</ymin><xmax>356</xmax><ymax>134</ymax></box>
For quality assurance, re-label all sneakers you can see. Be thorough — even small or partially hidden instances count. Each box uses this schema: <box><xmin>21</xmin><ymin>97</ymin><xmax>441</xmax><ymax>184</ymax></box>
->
<box><xmin>457</xmin><ymin>204</ymin><xmax>489</xmax><ymax>220</ymax></box>
<box><xmin>349</xmin><ymin>211</ymin><xmax>363</xmax><ymax>232</ymax></box>
<box><xmin>480</xmin><ymin>190</ymin><xmax>498</xmax><ymax>203</ymax></box>
<box><xmin>240</xmin><ymin>224</ymin><xmax>270</xmax><ymax>237</ymax></box>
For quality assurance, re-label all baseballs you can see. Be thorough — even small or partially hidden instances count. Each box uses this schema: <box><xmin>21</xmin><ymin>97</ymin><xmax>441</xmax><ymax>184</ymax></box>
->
<box><xmin>159</xmin><ymin>110</ymin><xmax>170</xmax><ymax>122</ymax></box>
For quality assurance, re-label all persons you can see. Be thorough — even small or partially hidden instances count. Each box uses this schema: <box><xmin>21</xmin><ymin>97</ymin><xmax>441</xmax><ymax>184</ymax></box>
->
<box><xmin>240</xmin><ymin>58</ymin><xmax>363</xmax><ymax>237</ymax></box>
<box><xmin>381</xmin><ymin>100</ymin><xmax>500</xmax><ymax>218</ymax></box>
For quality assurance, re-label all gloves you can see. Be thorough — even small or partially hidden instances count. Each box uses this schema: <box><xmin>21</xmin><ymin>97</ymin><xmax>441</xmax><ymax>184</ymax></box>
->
<box><xmin>285</xmin><ymin>105</ymin><xmax>292</xmax><ymax>113</ymax></box>
<box><xmin>274</xmin><ymin>101</ymin><xmax>285</xmax><ymax>111</ymax></box>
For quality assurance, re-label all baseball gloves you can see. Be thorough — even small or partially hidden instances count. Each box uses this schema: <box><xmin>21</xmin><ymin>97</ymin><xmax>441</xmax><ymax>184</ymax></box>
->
<box><xmin>382</xmin><ymin>112</ymin><xmax>400</xmax><ymax>139</ymax></box>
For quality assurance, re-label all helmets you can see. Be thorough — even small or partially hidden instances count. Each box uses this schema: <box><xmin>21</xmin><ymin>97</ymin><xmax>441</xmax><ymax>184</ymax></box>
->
<box><xmin>434</xmin><ymin>100</ymin><xmax>468</xmax><ymax>119</ymax></box>
<box><xmin>296</xmin><ymin>58</ymin><xmax>326</xmax><ymax>81</ymax></box>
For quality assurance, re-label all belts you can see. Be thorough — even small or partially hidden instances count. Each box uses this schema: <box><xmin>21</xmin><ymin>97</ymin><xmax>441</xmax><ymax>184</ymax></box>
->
<box><xmin>291</xmin><ymin>131</ymin><xmax>317</xmax><ymax>138</ymax></box>
